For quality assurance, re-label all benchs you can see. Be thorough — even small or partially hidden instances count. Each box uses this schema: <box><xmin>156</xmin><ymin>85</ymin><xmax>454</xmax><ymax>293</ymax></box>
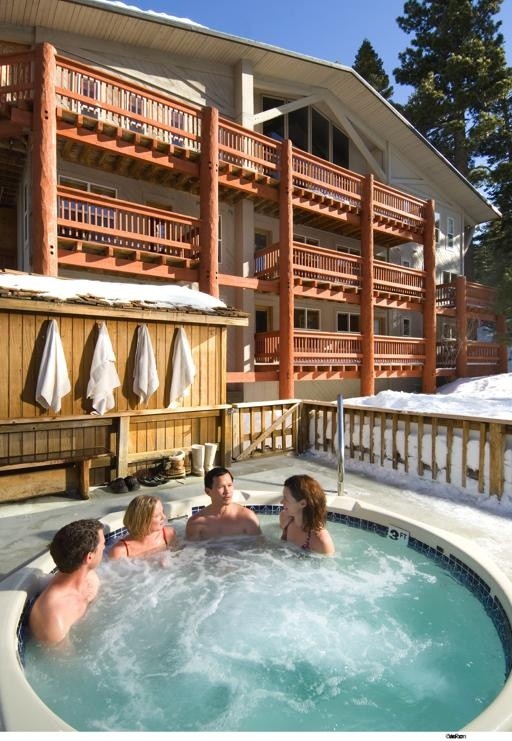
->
<box><xmin>0</xmin><ymin>445</ymin><xmax>118</xmax><ymax>502</ymax></box>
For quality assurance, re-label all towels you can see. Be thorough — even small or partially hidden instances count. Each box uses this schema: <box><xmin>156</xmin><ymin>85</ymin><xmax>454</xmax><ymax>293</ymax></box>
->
<box><xmin>34</xmin><ymin>320</ymin><xmax>199</xmax><ymax>416</ymax></box>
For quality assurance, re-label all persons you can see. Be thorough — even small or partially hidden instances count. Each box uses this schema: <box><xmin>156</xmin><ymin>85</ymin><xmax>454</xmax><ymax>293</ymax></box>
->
<box><xmin>185</xmin><ymin>469</ymin><xmax>263</xmax><ymax>547</ymax></box>
<box><xmin>277</xmin><ymin>475</ymin><xmax>333</xmax><ymax>555</ymax></box>
<box><xmin>109</xmin><ymin>496</ymin><xmax>178</xmax><ymax>572</ymax></box>
<box><xmin>29</xmin><ymin>520</ymin><xmax>107</xmax><ymax>648</ymax></box>
